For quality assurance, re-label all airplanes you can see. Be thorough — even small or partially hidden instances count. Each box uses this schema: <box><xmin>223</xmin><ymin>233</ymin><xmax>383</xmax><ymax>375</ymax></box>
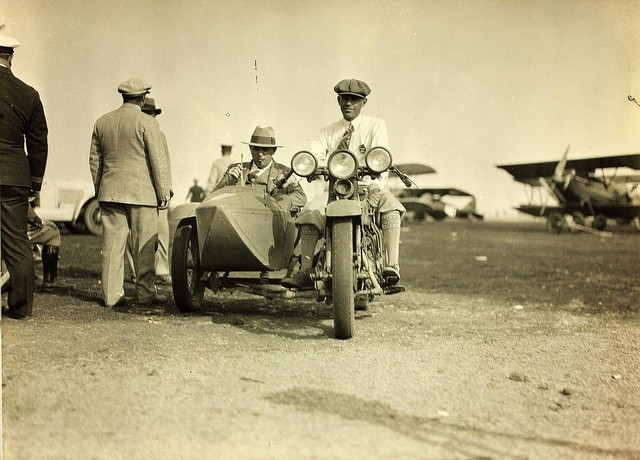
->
<box><xmin>495</xmin><ymin>152</ymin><xmax>640</xmax><ymax>236</ymax></box>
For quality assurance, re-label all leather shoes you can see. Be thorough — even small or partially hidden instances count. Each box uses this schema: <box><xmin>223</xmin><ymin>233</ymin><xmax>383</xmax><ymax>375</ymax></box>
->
<box><xmin>155</xmin><ymin>294</ymin><xmax>167</xmax><ymax>302</ymax></box>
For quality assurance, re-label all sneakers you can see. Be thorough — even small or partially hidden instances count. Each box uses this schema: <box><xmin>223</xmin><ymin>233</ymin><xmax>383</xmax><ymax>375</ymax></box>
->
<box><xmin>281</xmin><ymin>272</ymin><xmax>313</xmax><ymax>288</ymax></box>
<box><xmin>382</xmin><ymin>265</ymin><xmax>400</xmax><ymax>285</ymax></box>
<box><xmin>155</xmin><ymin>274</ymin><xmax>172</xmax><ymax>285</ymax></box>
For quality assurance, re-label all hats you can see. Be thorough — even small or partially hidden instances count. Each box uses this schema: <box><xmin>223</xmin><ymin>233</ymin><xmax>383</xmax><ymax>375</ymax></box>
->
<box><xmin>142</xmin><ymin>98</ymin><xmax>161</xmax><ymax>115</ymax></box>
<box><xmin>335</xmin><ymin>79</ymin><xmax>371</xmax><ymax>99</ymax></box>
<box><xmin>0</xmin><ymin>35</ymin><xmax>19</xmax><ymax>54</ymax></box>
<box><xmin>118</xmin><ymin>78</ymin><xmax>151</xmax><ymax>97</ymax></box>
<box><xmin>242</xmin><ymin>125</ymin><xmax>283</xmax><ymax>147</ymax></box>
<box><xmin>220</xmin><ymin>140</ymin><xmax>234</xmax><ymax>149</ymax></box>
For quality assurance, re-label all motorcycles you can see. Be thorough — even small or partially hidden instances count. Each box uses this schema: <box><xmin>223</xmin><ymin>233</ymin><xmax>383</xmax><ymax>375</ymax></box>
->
<box><xmin>168</xmin><ymin>147</ymin><xmax>422</xmax><ymax>339</ymax></box>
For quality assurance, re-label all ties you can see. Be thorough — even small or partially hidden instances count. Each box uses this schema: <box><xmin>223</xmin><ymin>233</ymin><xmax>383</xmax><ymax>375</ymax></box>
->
<box><xmin>245</xmin><ymin>170</ymin><xmax>259</xmax><ymax>184</ymax></box>
<box><xmin>337</xmin><ymin>123</ymin><xmax>353</xmax><ymax>149</ymax></box>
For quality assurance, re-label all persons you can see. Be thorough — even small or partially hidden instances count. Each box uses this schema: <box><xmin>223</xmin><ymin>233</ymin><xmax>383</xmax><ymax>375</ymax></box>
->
<box><xmin>283</xmin><ymin>78</ymin><xmax>406</xmax><ymax>287</ymax></box>
<box><xmin>25</xmin><ymin>201</ymin><xmax>62</xmax><ymax>288</ymax></box>
<box><xmin>1</xmin><ymin>34</ymin><xmax>47</xmax><ymax>319</ymax></box>
<box><xmin>187</xmin><ymin>179</ymin><xmax>204</xmax><ymax>203</ymax></box>
<box><xmin>127</xmin><ymin>97</ymin><xmax>172</xmax><ymax>286</ymax></box>
<box><xmin>207</xmin><ymin>143</ymin><xmax>233</xmax><ymax>192</ymax></box>
<box><xmin>88</xmin><ymin>79</ymin><xmax>170</xmax><ymax>308</ymax></box>
<box><xmin>212</xmin><ymin>124</ymin><xmax>308</xmax><ymax>214</ymax></box>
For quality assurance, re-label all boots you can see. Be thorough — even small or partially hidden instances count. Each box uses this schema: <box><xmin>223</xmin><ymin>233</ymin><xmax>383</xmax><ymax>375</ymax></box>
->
<box><xmin>42</xmin><ymin>245</ymin><xmax>76</xmax><ymax>291</ymax></box>
